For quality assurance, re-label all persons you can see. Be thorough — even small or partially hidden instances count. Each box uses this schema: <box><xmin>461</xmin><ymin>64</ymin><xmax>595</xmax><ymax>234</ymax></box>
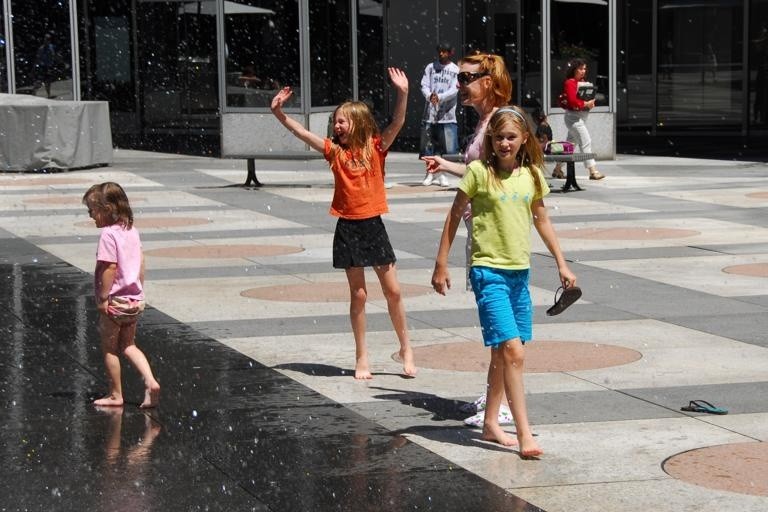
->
<box><xmin>527</xmin><ymin>106</ymin><xmax>552</xmax><ymax>156</ymax></box>
<box><xmin>269</xmin><ymin>66</ymin><xmax>418</xmax><ymax>381</ymax></box>
<box><xmin>420</xmin><ymin>49</ymin><xmax>515</xmax><ymax>430</ymax></box>
<box><xmin>550</xmin><ymin>57</ymin><xmax>608</xmax><ymax>181</ymax></box>
<box><xmin>79</xmin><ymin>180</ymin><xmax>162</xmax><ymax>409</ymax></box>
<box><xmin>420</xmin><ymin>40</ymin><xmax>461</xmax><ymax>186</ymax></box>
<box><xmin>429</xmin><ymin>104</ymin><xmax>576</xmax><ymax>459</ymax></box>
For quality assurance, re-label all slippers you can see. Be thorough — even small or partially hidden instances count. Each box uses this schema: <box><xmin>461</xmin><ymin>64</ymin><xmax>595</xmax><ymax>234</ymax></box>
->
<box><xmin>679</xmin><ymin>398</ymin><xmax>729</xmax><ymax>416</ymax></box>
<box><xmin>544</xmin><ymin>283</ymin><xmax>583</xmax><ymax>318</ymax></box>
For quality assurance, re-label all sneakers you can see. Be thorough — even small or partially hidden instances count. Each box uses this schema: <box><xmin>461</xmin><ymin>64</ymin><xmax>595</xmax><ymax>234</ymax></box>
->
<box><xmin>421</xmin><ymin>171</ymin><xmax>434</xmax><ymax>186</ymax></box>
<box><xmin>551</xmin><ymin>168</ymin><xmax>566</xmax><ymax>180</ymax></box>
<box><xmin>589</xmin><ymin>170</ymin><xmax>605</xmax><ymax>181</ymax></box>
<box><xmin>437</xmin><ymin>174</ymin><xmax>448</xmax><ymax>186</ymax></box>
<box><xmin>459</xmin><ymin>394</ymin><xmax>487</xmax><ymax>413</ymax></box>
<box><xmin>463</xmin><ymin>404</ymin><xmax>514</xmax><ymax>425</ymax></box>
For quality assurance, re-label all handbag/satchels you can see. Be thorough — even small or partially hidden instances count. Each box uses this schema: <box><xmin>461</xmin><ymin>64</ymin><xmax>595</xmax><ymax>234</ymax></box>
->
<box><xmin>557</xmin><ymin>93</ymin><xmax>575</xmax><ymax>110</ymax></box>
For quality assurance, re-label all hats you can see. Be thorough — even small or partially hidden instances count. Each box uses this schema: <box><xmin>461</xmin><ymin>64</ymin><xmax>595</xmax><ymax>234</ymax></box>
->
<box><xmin>436</xmin><ymin>43</ymin><xmax>451</xmax><ymax>52</ymax></box>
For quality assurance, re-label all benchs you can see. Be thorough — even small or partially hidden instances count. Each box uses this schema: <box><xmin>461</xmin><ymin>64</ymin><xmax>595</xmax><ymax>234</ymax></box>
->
<box><xmin>441</xmin><ymin>152</ymin><xmax>596</xmax><ymax>191</ymax></box>
<box><xmin>225</xmin><ymin>150</ymin><xmax>331</xmax><ymax>188</ymax></box>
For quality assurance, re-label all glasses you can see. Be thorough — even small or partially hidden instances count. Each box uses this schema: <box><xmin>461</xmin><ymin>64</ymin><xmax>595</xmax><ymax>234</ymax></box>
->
<box><xmin>455</xmin><ymin>71</ymin><xmax>491</xmax><ymax>86</ymax></box>
